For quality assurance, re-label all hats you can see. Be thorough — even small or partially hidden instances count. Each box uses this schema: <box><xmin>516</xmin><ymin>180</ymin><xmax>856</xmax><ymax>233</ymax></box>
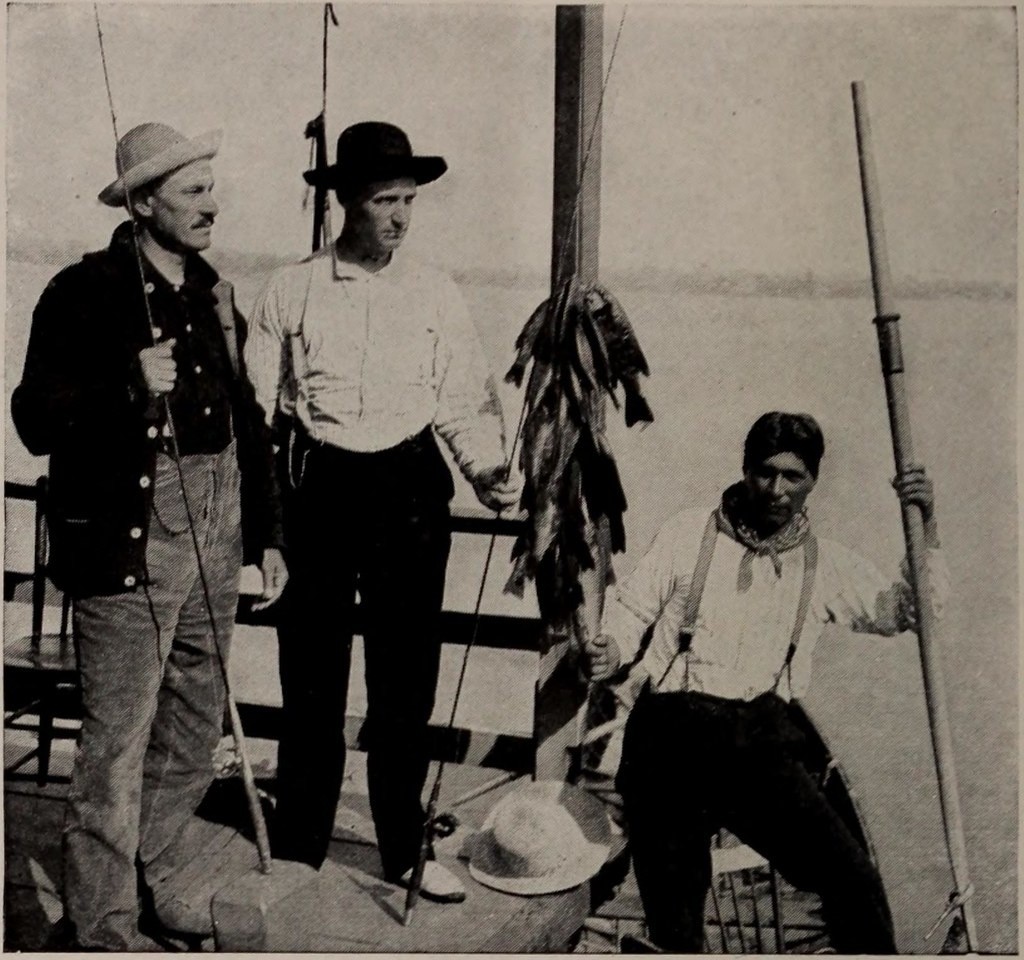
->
<box><xmin>469</xmin><ymin>778</ymin><xmax>628</xmax><ymax>895</ymax></box>
<box><xmin>98</xmin><ymin>122</ymin><xmax>222</xmax><ymax>207</ymax></box>
<box><xmin>302</xmin><ymin>121</ymin><xmax>448</xmax><ymax>186</ymax></box>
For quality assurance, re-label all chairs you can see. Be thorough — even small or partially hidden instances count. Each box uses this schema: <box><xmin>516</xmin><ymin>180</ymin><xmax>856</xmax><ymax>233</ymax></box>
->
<box><xmin>3</xmin><ymin>472</ymin><xmax>85</xmax><ymax>788</ymax></box>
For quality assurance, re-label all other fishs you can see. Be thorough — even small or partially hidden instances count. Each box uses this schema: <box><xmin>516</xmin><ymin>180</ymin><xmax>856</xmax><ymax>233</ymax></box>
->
<box><xmin>501</xmin><ymin>281</ymin><xmax>655</xmax><ymax>642</ymax></box>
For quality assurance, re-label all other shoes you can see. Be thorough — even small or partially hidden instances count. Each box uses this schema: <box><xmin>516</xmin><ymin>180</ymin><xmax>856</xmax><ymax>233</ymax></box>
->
<box><xmin>161</xmin><ymin>903</ymin><xmax>213</xmax><ymax>937</ymax></box>
<box><xmin>401</xmin><ymin>857</ymin><xmax>468</xmax><ymax>900</ymax></box>
<box><xmin>80</xmin><ymin>933</ymin><xmax>165</xmax><ymax>952</ymax></box>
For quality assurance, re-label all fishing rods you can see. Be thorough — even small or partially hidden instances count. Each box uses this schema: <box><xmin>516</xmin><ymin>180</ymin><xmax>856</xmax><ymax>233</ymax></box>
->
<box><xmin>90</xmin><ymin>1</ymin><xmax>274</xmax><ymax>870</ymax></box>
<box><xmin>402</xmin><ymin>1</ymin><xmax>629</xmax><ymax>926</ymax></box>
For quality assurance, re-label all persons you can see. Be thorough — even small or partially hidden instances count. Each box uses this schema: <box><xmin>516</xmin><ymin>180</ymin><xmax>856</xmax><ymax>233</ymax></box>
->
<box><xmin>244</xmin><ymin>123</ymin><xmax>526</xmax><ymax>903</ymax></box>
<box><xmin>584</xmin><ymin>409</ymin><xmax>949</xmax><ymax>956</ymax></box>
<box><xmin>11</xmin><ymin>120</ymin><xmax>289</xmax><ymax>953</ymax></box>
